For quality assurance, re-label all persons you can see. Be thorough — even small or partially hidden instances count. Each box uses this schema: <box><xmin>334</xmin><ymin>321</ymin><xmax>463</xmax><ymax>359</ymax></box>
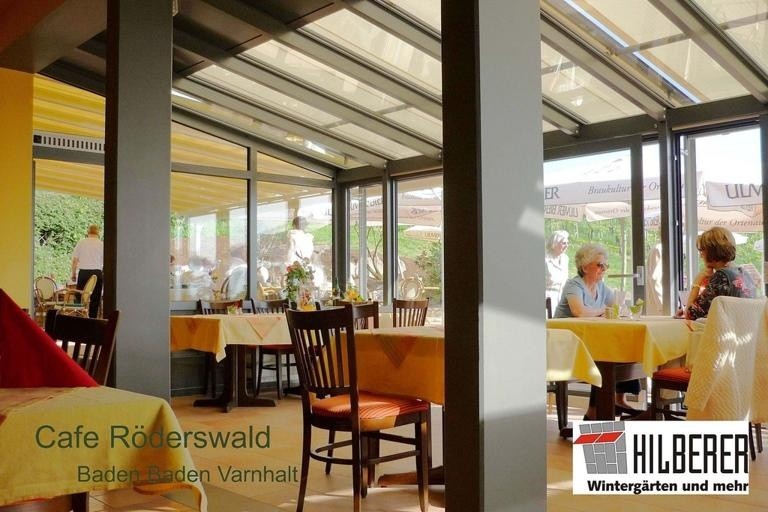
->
<box><xmin>543</xmin><ymin>231</ymin><xmax>571</xmax><ymax>316</ymax></box>
<box><xmin>71</xmin><ymin>225</ymin><xmax>104</xmax><ymax>318</ymax></box>
<box><xmin>553</xmin><ymin>243</ymin><xmax>639</xmax><ymax>421</ymax></box>
<box><xmin>647</xmin><ymin>226</ymin><xmax>664</xmax><ymax>314</ymax></box>
<box><xmin>675</xmin><ymin>225</ymin><xmax>765</xmax><ymax>321</ymax></box>
<box><xmin>286</xmin><ymin>215</ymin><xmax>315</xmax><ymax>268</ymax></box>
<box><xmin>226</xmin><ymin>242</ymin><xmax>248</xmax><ymax>281</ymax></box>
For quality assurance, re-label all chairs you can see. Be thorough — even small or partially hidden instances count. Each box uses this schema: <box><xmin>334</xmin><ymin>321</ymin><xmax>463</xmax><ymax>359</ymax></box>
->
<box><xmin>33</xmin><ymin>274</ymin><xmax>121</xmax><ymax>387</ymax></box>
<box><xmin>651</xmin><ymin>296</ymin><xmax>768</xmax><ymax>460</ymax></box>
<box><xmin>221</xmin><ymin>263</ymin><xmax>280</xmax><ymax>297</ymax></box>
<box><xmin>198</xmin><ymin>299</ymin><xmax>257</xmax><ymax>399</ymax></box>
<box><xmin>253</xmin><ymin>298</ymin><xmax>300</xmax><ymax>400</ymax></box>
<box><xmin>313</xmin><ymin>298</ymin><xmax>430</xmax><ymax>346</ymax></box>
<box><xmin>546</xmin><ymin>296</ymin><xmax>568</xmax><ymax>430</ymax></box>
<box><xmin>283</xmin><ymin>302</ymin><xmax>432</xmax><ymax>511</ymax></box>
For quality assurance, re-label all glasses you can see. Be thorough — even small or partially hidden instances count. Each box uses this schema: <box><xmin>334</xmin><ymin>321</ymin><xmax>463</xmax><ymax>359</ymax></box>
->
<box><xmin>593</xmin><ymin>262</ymin><xmax>610</xmax><ymax>270</ymax></box>
<box><xmin>561</xmin><ymin>241</ymin><xmax>569</xmax><ymax>245</ymax></box>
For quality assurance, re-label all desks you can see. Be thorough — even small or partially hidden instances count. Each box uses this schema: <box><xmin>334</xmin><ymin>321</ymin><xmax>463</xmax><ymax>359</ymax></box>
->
<box><xmin>0</xmin><ymin>385</ymin><xmax>208</xmax><ymax>511</ymax></box>
<box><xmin>170</xmin><ymin>313</ymin><xmax>293</xmax><ymax>413</ymax></box>
<box><xmin>309</xmin><ymin>325</ymin><xmax>603</xmax><ymax>489</ymax></box>
<box><xmin>545</xmin><ymin>311</ymin><xmax>699</xmax><ymax>420</ymax></box>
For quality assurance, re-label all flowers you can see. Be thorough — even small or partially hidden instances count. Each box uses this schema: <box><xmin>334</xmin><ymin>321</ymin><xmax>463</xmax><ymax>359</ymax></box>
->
<box><xmin>283</xmin><ymin>260</ymin><xmax>314</xmax><ymax>299</ymax></box>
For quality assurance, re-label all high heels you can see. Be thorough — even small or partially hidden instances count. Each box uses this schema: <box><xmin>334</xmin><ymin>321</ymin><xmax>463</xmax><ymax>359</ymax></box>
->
<box><xmin>614</xmin><ymin>402</ymin><xmax>646</xmax><ymax>416</ymax></box>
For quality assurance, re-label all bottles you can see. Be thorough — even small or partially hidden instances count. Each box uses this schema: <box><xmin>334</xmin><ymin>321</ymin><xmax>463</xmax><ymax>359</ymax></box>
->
<box><xmin>227</xmin><ymin>306</ymin><xmax>243</xmax><ymax>315</ymax></box>
<box><xmin>603</xmin><ymin>303</ymin><xmax>641</xmax><ymax>320</ymax></box>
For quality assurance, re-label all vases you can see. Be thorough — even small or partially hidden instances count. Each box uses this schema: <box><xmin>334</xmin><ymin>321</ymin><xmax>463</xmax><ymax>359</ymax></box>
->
<box><xmin>287</xmin><ymin>294</ymin><xmax>300</xmax><ymax>310</ymax></box>
